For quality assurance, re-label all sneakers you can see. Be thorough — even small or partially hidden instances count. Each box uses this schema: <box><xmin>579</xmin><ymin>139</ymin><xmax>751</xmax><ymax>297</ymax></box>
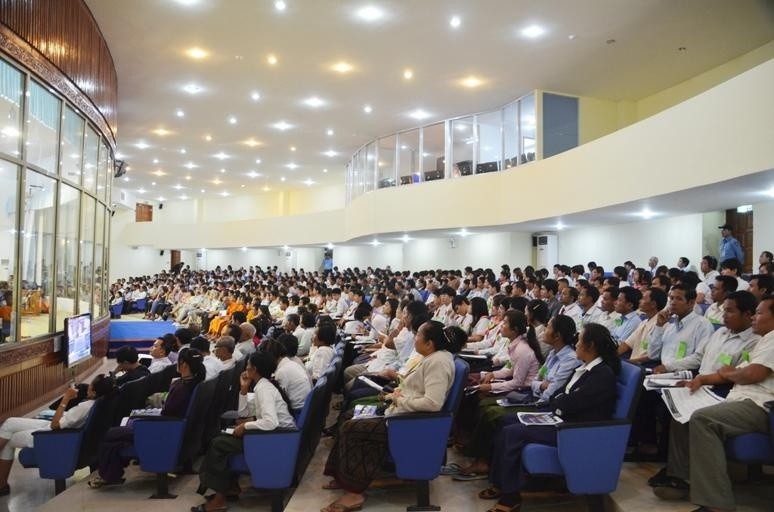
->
<box><xmin>88</xmin><ymin>476</ymin><xmax>121</xmax><ymax>489</ymax></box>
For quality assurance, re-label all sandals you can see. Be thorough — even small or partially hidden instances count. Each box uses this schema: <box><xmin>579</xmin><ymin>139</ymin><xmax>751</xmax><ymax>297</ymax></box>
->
<box><xmin>439</xmin><ymin>462</ymin><xmax>523</xmax><ymax>512</ymax></box>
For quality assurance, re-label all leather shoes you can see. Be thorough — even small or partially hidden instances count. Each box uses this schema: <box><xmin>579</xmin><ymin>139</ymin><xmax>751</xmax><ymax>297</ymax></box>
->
<box><xmin>623</xmin><ymin>447</ymin><xmax>735</xmax><ymax>512</ymax></box>
<box><xmin>322</xmin><ymin>424</ymin><xmax>338</xmax><ymax>436</ymax></box>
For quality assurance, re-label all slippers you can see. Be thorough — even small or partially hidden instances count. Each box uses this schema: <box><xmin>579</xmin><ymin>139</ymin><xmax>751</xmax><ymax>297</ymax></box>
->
<box><xmin>320</xmin><ymin>492</ymin><xmax>368</xmax><ymax>512</ymax></box>
<box><xmin>191</xmin><ymin>504</ymin><xmax>228</xmax><ymax>512</ymax></box>
<box><xmin>204</xmin><ymin>494</ymin><xmax>239</xmax><ymax>502</ymax></box>
<box><xmin>322</xmin><ymin>480</ymin><xmax>345</xmax><ymax>489</ymax></box>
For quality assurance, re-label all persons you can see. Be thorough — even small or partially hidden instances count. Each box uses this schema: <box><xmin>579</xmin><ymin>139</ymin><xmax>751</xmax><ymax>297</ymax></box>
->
<box><xmin>718</xmin><ymin>224</ymin><xmax>744</xmax><ymax>268</ymax></box>
<box><xmin>1</xmin><ymin>253</ymin><xmax>774</xmax><ymax>512</ymax></box>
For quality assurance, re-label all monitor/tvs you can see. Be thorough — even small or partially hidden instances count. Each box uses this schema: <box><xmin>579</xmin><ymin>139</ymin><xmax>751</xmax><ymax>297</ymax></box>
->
<box><xmin>64</xmin><ymin>312</ymin><xmax>93</xmax><ymax>369</ymax></box>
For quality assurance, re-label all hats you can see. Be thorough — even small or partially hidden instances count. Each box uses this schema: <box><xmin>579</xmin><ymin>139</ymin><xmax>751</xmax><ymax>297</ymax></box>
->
<box><xmin>718</xmin><ymin>224</ymin><xmax>733</xmax><ymax>231</ymax></box>
